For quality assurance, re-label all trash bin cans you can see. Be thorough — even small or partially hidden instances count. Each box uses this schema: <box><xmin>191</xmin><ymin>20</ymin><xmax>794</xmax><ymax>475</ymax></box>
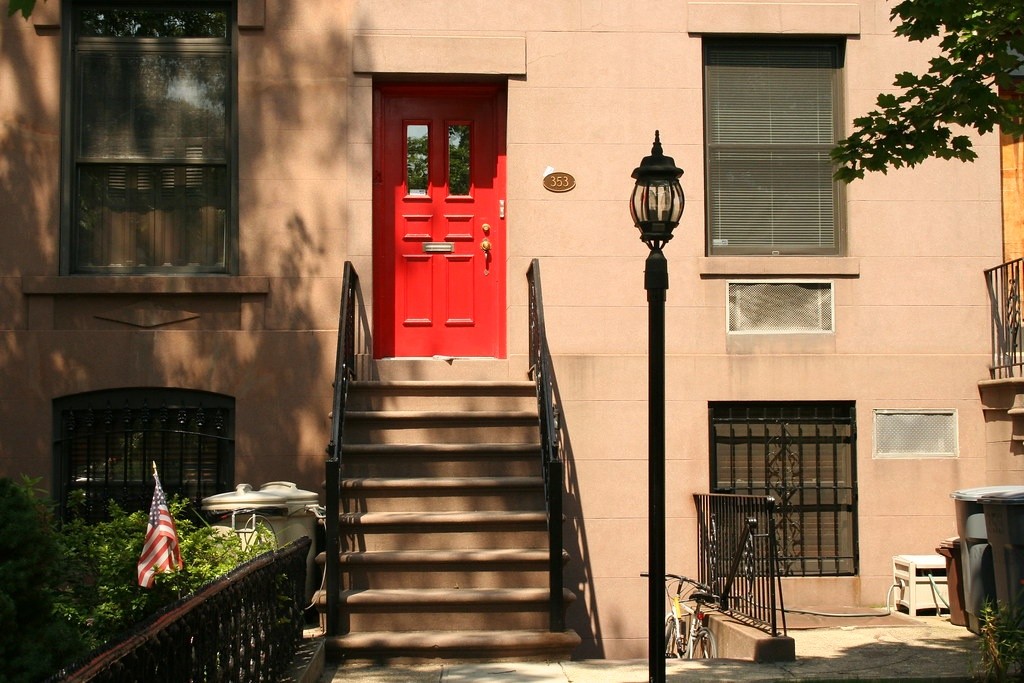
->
<box><xmin>200</xmin><ymin>479</ymin><xmax>320</xmax><ymax>605</ymax></box>
<box><xmin>935</xmin><ymin>537</ymin><xmax>969</xmax><ymax>627</ymax></box>
<box><xmin>949</xmin><ymin>485</ymin><xmax>1024</xmax><ymax>635</ymax></box>
<box><xmin>977</xmin><ymin>490</ymin><xmax>1024</xmax><ymax>617</ymax></box>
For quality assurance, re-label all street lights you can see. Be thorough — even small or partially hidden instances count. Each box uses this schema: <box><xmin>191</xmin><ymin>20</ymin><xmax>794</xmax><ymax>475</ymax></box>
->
<box><xmin>623</xmin><ymin>129</ymin><xmax>686</xmax><ymax>683</ymax></box>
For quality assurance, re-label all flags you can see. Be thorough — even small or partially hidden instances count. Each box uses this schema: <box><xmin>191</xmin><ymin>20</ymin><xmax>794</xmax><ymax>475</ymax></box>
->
<box><xmin>136</xmin><ymin>473</ymin><xmax>183</xmax><ymax>588</ymax></box>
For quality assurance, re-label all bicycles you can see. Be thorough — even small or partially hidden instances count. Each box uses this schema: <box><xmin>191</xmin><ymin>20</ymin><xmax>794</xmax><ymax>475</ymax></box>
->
<box><xmin>638</xmin><ymin>572</ymin><xmax>718</xmax><ymax>660</ymax></box>
